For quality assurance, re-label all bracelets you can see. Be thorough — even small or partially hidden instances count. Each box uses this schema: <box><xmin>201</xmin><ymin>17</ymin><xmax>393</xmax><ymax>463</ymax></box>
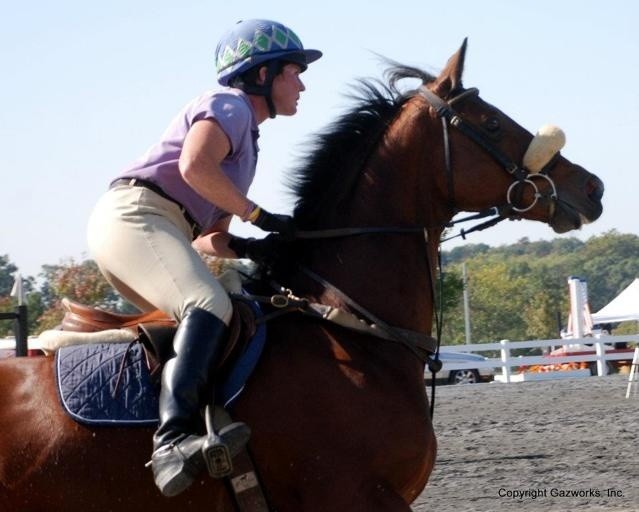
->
<box><xmin>240</xmin><ymin>203</ymin><xmax>264</xmax><ymax>225</ymax></box>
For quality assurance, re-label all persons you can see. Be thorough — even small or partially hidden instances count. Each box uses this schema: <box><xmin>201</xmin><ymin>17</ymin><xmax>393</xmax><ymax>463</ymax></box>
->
<box><xmin>84</xmin><ymin>19</ymin><xmax>324</xmax><ymax>499</ymax></box>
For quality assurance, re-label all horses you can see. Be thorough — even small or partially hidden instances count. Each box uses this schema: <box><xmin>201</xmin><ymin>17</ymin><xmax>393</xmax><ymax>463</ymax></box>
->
<box><xmin>0</xmin><ymin>35</ymin><xmax>609</xmax><ymax>512</ymax></box>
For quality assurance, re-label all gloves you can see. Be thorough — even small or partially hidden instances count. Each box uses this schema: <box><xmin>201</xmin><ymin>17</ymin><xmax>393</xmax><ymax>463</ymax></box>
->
<box><xmin>228</xmin><ymin>233</ymin><xmax>280</xmax><ymax>271</ymax></box>
<box><xmin>252</xmin><ymin>208</ymin><xmax>293</xmax><ymax>244</ymax></box>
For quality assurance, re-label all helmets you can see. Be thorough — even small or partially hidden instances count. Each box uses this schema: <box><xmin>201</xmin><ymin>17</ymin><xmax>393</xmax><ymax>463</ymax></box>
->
<box><xmin>216</xmin><ymin>19</ymin><xmax>322</xmax><ymax>86</ymax></box>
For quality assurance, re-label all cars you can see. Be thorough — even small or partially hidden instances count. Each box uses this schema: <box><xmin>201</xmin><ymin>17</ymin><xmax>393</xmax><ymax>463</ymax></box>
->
<box><xmin>424</xmin><ymin>352</ymin><xmax>496</xmax><ymax>385</ymax></box>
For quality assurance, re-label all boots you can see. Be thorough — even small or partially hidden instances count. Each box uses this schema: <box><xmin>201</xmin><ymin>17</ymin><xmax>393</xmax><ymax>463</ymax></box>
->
<box><xmin>152</xmin><ymin>305</ymin><xmax>251</xmax><ymax>497</ymax></box>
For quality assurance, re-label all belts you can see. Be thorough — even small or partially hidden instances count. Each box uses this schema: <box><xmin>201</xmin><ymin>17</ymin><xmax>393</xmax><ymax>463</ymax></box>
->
<box><xmin>110</xmin><ymin>178</ymin><xmax>201</xmax><ymax>241</ymax></box>
